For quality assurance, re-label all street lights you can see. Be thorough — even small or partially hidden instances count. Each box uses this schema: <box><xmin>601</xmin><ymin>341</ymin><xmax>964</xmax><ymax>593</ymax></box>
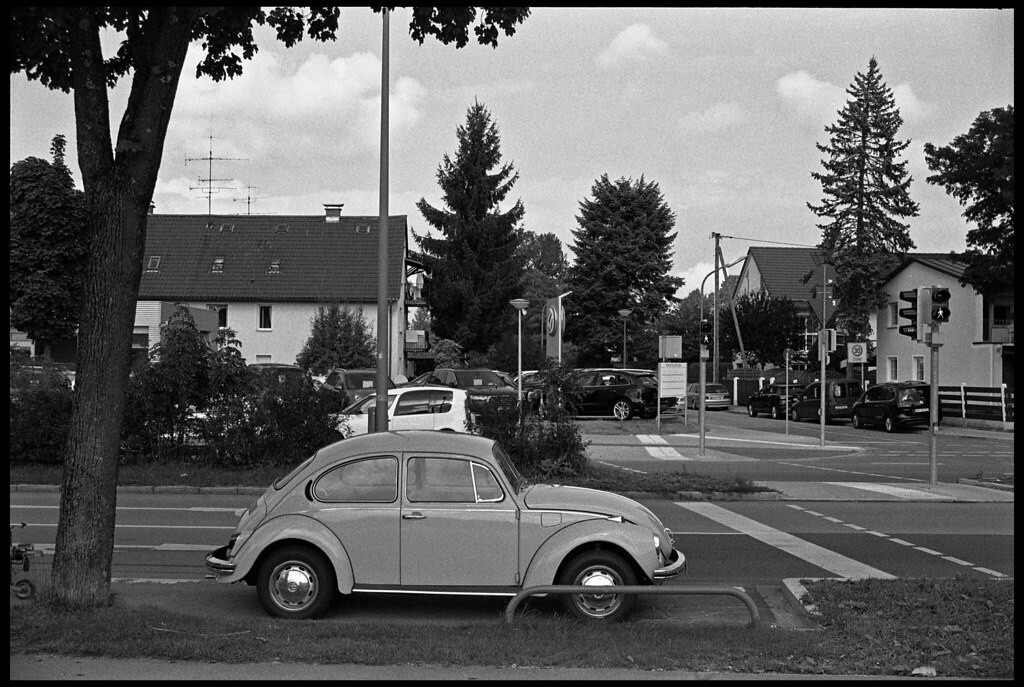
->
<box><xmin>700</xmin><ymin>255</ymin><xmax>750</xmax><ymax>457</ymax></box>
<box><xmin>507</xmin><ymin>297</ymin><xmax>530</xmax><ymax>426</ymax></box>
<box><xmin>618</xmin><ymin>307</ymin><xmax>633</xmax><ymax>370</ymax></box>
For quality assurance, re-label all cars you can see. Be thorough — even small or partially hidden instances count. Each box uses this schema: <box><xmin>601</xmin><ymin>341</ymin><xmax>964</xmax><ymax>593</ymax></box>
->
<box><xmin>408</xmin><ymin>370</ymin><xmax>526</xmax><ymax>419</ymax></box>
<box><xmin>509</xmin><ymin>369</ymin><xmax>543</xmax><ymax>399</ymax></box>
<box><xmin>204</xmin><ymin>428</ymin><xmax>687</xmax><ymax>621</ymax></box>
<box><xmin>323</xmin><ymin>386</ymin><xmax>483</xmax><ymax>438</ymax></box>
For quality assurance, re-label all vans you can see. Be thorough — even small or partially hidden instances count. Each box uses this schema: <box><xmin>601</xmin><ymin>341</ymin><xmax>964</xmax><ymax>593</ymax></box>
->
<box><xmin>248</xmin><ymin>363</ymin><xmax>313</xmax><ymax>402</ymax></box>
<box><xmin>540</xmin><ymin>368</ymin><xmax>676</xmax><ymax>421</ymax></box>
<box><xmin>686</xmin><ymin>382</ymin><xmax>731</xmax><ymax>411</ymax></box>
<box><xmin>790</xmin><ymin>378</ymin><xmax>863</xmax><ymax>424</ymax></box>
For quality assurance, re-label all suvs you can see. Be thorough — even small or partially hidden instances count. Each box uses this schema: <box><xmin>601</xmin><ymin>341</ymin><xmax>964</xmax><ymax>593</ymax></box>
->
<box><xmin>748</xmin><ymin>384</ymin><xmax>805</xmax><ymax>418</ymax></box>
<box><xmin>852</xmin><ymin>379</ymin><xmax>943</xmax><ymax>434</ymax></box>
<box><xmin>318</xmin><ymin>367</ymin><xmax>395</xmax><ymax>406</ymax></box>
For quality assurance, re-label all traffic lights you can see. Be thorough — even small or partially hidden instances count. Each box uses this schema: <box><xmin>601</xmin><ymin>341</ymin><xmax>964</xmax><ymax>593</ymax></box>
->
<box><xmin>826</xmin><ymin>329</ymin><xmax>837</xmax><ymax>353</ymax></box>
<box><xmin>899</xmin><ymin>289</ymin><xmax>917</xmax><ymax>338</ymax></box>
<box><xmin>933</xmin><ymin>287</ymin><xmax>951</xmax><ymax>322</ymax></box>
<box><xmin>700</xmin><ymin>321</ymin><xmax>711</xmax><ymax>344</ymax></box>
